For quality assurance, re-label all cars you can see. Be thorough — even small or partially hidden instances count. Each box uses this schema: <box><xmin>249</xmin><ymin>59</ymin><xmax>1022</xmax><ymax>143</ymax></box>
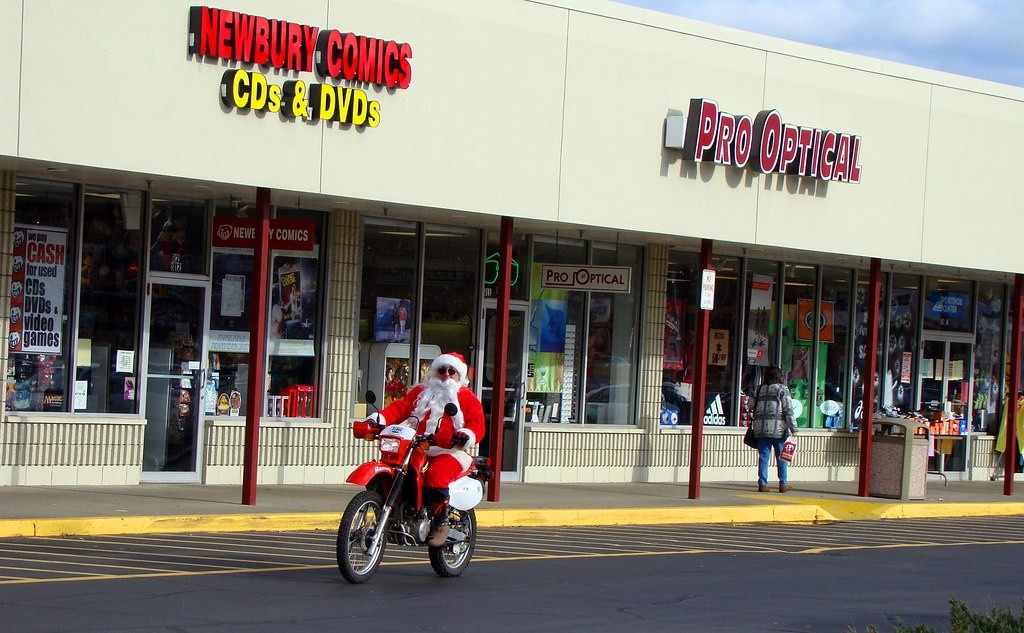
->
<box><xmin>583</xmin><ymin>384</ymin><xmax>667</xmax><ymax>426</ymax></box>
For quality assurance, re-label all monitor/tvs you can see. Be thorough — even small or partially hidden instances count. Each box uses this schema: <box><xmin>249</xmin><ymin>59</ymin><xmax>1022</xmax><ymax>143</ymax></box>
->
<box><xmin>923</xmin><ymin>280</ymin><xmax>979</xmax><ymax>344</ymax></box>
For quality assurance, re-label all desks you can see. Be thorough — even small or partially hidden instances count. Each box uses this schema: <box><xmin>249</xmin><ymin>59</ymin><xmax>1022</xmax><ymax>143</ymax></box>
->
<box><xmin>890</xmin><ymin>434</ymin><xmax>964</xmax><ymax>488</ymax></box>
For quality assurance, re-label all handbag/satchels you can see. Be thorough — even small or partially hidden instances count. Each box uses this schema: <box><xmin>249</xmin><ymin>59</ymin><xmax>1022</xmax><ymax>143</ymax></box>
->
<box><xmin>779</xmin><ymin>435</ymin><xmax>797</xmax><ymax>462</ymax></box>
<box><xmin>744</xmin><ymin>427</ymin><xmax>758</xmax><ymax>449</ymax></box>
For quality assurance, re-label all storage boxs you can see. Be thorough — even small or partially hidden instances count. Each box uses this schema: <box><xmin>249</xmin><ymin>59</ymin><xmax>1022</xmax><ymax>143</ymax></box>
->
<box><xmin>739</xmin><ymin>395</ymin><xmax>754</xmax><ymax>427</ymax></box>
<box><xmin>266</xmin><ymin>385</ymin><xmax>314</xmax><ymax>418</ymax></box>
<box><xmin>914</xmin><ymin>412</ymin><xmax>966</xmax><ymax>435</ymax></box>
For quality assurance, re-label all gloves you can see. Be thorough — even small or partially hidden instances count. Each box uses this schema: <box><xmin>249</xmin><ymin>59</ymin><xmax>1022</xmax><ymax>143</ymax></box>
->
<box><xmin>363</xmin><ymin>419</ymin><xmax>375</xmax><ymax>428</ymax></box>
<box><xmin>450</xmin><ymin>431</ymin><xmax>470</xmax><ymax>448</ymax></box>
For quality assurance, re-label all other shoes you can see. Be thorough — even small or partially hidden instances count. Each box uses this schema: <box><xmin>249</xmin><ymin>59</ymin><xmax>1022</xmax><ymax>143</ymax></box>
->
<box><xmin>757</xmin><ymin>485</ymin><xmax>771</xmax><ymax>492</ymax></box>
<box><xmin>426</xmin><ymin>508</ymin><xmax>451</xmax><ymax>548</ymax></box>
<box><xmin>778</xmin><ymin>485</ymin><xmax>793</xmax><ymax>493</ymax></box>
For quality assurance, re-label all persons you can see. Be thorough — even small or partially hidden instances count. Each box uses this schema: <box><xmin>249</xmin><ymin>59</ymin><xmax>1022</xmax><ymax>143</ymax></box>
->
<box><xmin>290</xmin><ymin>284</ymin><xmax>300</xmax><ymax>320</ymax></box>
<box><xmin>391</xmin><ymin>301</ymin><xmax>407</xmax><ymax>342</ymax></box>
<box><xmin>747</xmin><ymin>366</ymin><xmax>800</xmax><ymax>492</ymax></box>
<box><xmin>360</xmin><ymin>350</ymin><xmax>486</xmax><ymax>547</ymax></box>
<box><xmin>682</xmin><ymin>327</ymin><xmax>702</xmax><ymax>383</ymax></box>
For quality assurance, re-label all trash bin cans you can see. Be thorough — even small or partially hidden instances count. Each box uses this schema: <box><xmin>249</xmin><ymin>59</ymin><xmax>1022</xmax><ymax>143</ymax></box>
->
<box><xmin>869</xmin><ymin>419</ymin><xmax>930</xmax><ymax>501</ymax></box>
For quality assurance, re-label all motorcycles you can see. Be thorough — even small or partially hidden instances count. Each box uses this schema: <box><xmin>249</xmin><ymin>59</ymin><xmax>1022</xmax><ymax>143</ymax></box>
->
<box><xmin>335</xmin><ymin>390</ymin><xmax>495</xmax><ymax>584</ymax></box>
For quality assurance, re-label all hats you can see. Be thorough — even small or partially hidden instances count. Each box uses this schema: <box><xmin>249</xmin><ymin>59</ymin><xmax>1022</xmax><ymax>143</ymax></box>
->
<box><xmin>431</xmin><ymin>352</ymin><xmax>468</xmax><ymax>382</ymax></box>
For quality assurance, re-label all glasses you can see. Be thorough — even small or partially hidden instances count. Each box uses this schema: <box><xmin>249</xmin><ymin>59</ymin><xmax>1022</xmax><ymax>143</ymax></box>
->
<box><xmin>437</xmin><ymin>367</ymin><xmax>456</xmax><ymax>375</ymax></box>
